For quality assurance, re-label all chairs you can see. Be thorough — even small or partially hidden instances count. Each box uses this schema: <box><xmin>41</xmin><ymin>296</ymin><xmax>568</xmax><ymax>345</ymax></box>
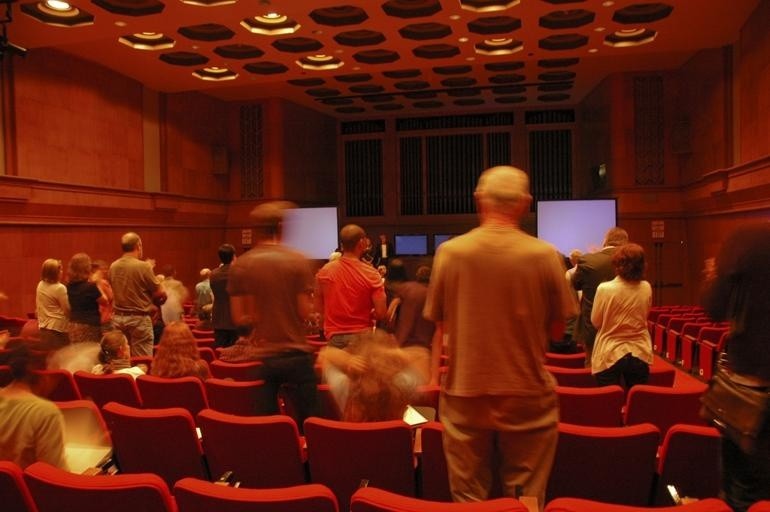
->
<box><xmin>7</xmin><ymin>304</ymin><xmax>769</xmax><ymax>512</ymax></box>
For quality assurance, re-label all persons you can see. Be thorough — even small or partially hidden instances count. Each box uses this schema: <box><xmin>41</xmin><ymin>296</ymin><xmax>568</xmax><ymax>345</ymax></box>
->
<box><xmin>588</xmin><ymin>242</ymin><xmax>654</xmax><ymax>387</ymax></box>
<box><xmin>422</xmin><ymin>164</ymin><xmax>585</xmax><ymax>511</ymax></box>
<box><xmin>36</xmin><ymin>231</ymin><xmax>241</xmax><ymax>379</ymax></box>
<box><xmin>0</xmin><ymin>337</ymin><xmax>71</xmax><ymax>474</ymax></box>
<box><xmin>329</xmin><ymin>225</ymin><xmax>635</xmax><ymax>352</ymax></box>
<box><xmin>312</xmin><ymin>222</ymin><xmax>389</xmax><ymax>347</ymax></box>
<box><xmin>226</xmin><ymin>201</ymin><xmax>325</xmax><ymax>435</ymax></box>
<box><xmin>316</xmin><ymin>328</ymin><xmax>434</xmax><ymax>428</ymax></box>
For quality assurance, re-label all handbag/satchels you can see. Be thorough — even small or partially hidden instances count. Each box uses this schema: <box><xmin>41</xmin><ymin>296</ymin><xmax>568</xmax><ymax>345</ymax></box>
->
<box><xmin>700</xmin><ymin>367</ymin><xmax>770</xmax><ymax>454</ymax></box>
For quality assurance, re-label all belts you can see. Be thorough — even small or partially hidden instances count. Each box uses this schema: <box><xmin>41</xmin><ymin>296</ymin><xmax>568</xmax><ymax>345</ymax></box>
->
<box><xmin>114</xmin><ymin>309</ymin><xmax>149</xmax><ymax>315</ymax></box>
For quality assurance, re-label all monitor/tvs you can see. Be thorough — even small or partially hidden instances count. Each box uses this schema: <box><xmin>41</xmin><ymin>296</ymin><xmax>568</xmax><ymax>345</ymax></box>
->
<box><xmin>432</xmin><ymin>233</ymin><xmax>464</xmax><ymax>255</ymax></box>
<box><xmin>394</xmin><ymin>234</ymin><xmax>428</xmax><ymax>256</ymax></box>
